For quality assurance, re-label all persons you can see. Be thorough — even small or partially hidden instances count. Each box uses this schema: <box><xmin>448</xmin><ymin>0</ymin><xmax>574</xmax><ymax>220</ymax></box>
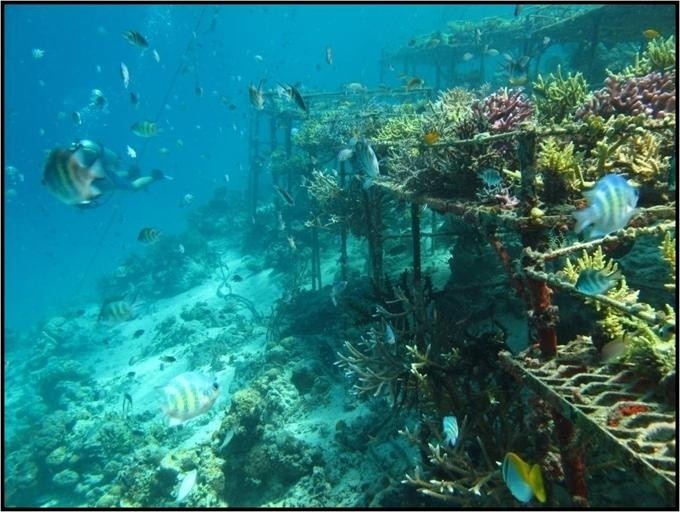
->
<box><xmin>66</xmin><ymin>138</ymin><xmax>163</xmax><ymax>192</ymax></box>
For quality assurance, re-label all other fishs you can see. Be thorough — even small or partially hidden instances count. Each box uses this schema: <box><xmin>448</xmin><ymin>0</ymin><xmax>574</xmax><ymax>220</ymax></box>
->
<box><xmin>4</xmin><ymin>0</ymin><xmax>677</xmax><ymax>243</ymax></box>
